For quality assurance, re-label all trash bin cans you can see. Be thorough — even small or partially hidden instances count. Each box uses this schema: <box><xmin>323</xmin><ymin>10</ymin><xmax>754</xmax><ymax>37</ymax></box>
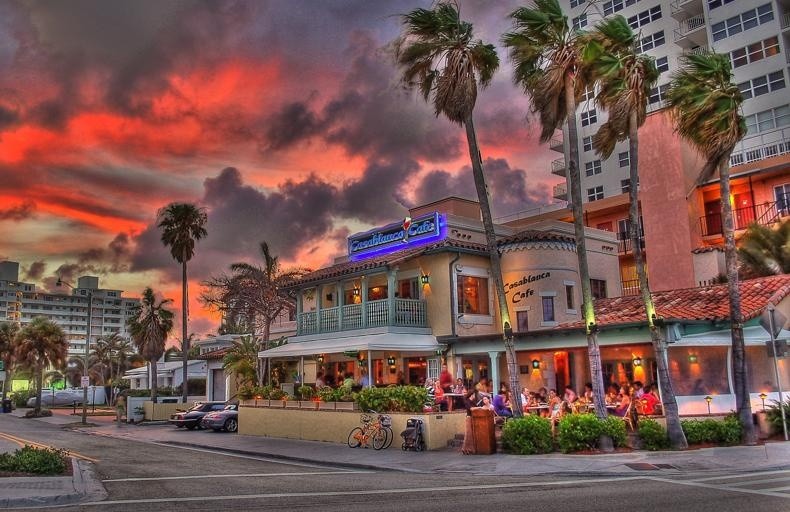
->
<box><xmin>2</xmin><ymin>398</ymin><xmax>12</xmax><ymax>413</ymax></box>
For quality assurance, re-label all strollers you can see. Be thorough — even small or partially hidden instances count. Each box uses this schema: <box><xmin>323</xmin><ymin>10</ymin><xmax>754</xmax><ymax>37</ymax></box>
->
<box><xmin>401</xmin><ymin>418</ymin><xmax>423</xmax><ymax>452</ymax></box>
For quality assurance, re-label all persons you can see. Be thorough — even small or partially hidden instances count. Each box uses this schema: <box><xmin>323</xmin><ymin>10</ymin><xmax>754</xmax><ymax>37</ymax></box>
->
<box><xmin>690</xmin><ymin>378</ymin><xmax>709</xmax><ymax>395</ymax></box>
<box><xmin>416</xmin><ymin>375</ymin><xmax>661</xmax><ymax>426</ymax></box>
<box><xmin>357</xmin><ymin>292</ymin><xmax>421</xmax><ymax>327</ymax></box>
<box><xmin>465</xmin><ymin>299</ymin><xmax>474</xmax><ymax>312</ymax></box>
<box><xmin>343</xmin><ymin>373</ymin><xmax>359</xmax><ymax>393</ymax></box>
<box><xmin>358</xmin><ymin>370</ymin><xmax>369</xmax><ymax>391</ymax></box>
<box><xmin>718</xmin><ymin>376</ymin><xmax>731</xmax><ymax>394</ymax></box>
<box><xmin>764</xmin><ymin>380</ymin><xmax>780</xmax><ymax>392</ymax></box>
<box><xmin>315</xmin><ymin>371</ymin><xmax>327</xmax><ymax>392</ymax></box>
<box><xmin>440</xmin><ymin>363</ymin><xmax>453</xmax><ymax>393</ymax></box>
<box><xmin>115</xmin><ymin>396</ymin><xmax>128</xmax><ymax>427</ymax></box>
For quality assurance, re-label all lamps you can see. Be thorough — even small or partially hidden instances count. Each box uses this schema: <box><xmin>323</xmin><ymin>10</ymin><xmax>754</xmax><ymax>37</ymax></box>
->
<box><xmin>317</xmin><ymin>355</ymin><xmax>323</xmax><ymax>362</ymax></box>
<box><xmin>632</xmin><ymin>357</ymin><xmax>641</xmax><ymax>366</ymax></box>
<box><xmin>353</xmin><ymin>288</ymin><xmax>359</xmax><ymax>296</ymax></box>
<box><xmin>421</xmin><ymin>275</ymin><xmax>429</xmax><ymax>283</ymax></box>
<box><xmin>388</xmin><ymin>356</ymin><xmax>395</xmax><ymax>366</ymax></box>
<box><xmin>359</xmin><ymin>359</ymin><xmax>365</xmax><ymax>365</ymax></box>
<box><xmin>532</xmin><ymin>359</ymin><xmax>539</xmax><ymax>369</ymax></box>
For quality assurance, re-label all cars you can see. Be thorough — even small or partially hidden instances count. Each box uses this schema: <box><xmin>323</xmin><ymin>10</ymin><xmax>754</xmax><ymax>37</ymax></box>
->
<box><xmin>170</xmin><ymin>401</ymin><xmax>238</xmax><ymax>432</ymax></box>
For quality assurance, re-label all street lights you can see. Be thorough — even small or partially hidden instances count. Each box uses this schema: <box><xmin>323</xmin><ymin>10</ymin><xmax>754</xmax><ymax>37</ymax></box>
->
<box><xmin>56</xmin><ymin>278</ymin><xmax>92</xmax><ymax>423</ymax></box>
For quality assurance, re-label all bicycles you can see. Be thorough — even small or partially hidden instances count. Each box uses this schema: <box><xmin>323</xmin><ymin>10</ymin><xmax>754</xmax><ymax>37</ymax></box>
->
<box><xmin>347</xmin><ymin>410</ymin><xmax>393</xmax><ymax>450</ymax></box>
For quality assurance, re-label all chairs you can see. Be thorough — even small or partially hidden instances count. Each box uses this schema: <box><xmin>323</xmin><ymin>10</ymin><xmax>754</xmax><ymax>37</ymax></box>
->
<box><xmin>623</xmin><ymin>397</ymin><xmax>643</xmax><ymax>431</ymax></box>
<box><xmin>548</xmin><ymin>401</ymin><xmax>565</xmax><ymax>438</ymax></box>
<box><xmin>494</xmin><ymin>411</ymin><xmax>507</xmax><ymax>425</ymax></box>
